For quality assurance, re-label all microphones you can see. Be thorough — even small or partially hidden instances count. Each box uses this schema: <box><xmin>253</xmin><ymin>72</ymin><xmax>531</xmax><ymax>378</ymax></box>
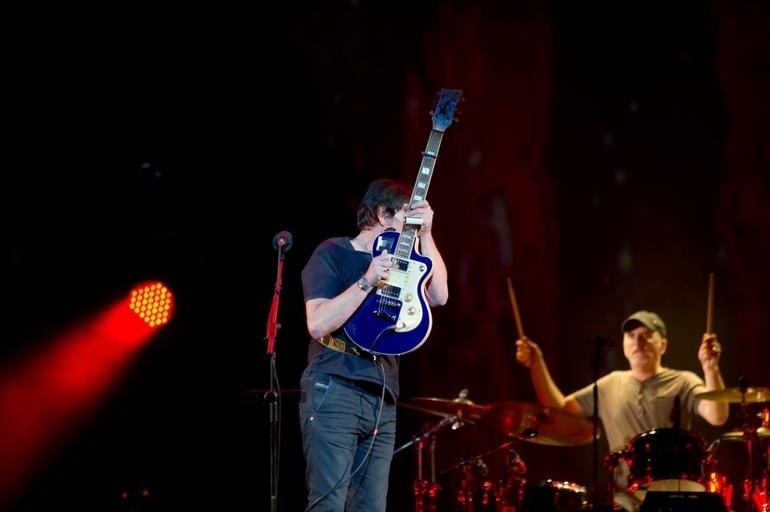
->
<box><xmin>271</xmin><ymin>230</ymin><xmax>293</xmax><ymax>254</ymax></box>
<box><xmin>590</xmin><ymin>335</ymin><xmax>611</xmax><ymax>384</ymax></box>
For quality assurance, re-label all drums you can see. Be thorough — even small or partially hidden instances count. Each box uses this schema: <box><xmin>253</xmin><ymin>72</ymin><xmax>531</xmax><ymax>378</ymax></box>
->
<box><xmin>622</xmin><ymin>427</ymin><xmax>707</xmax><ymax>505</ymax></box>
<box><xmin>521</xmin><ymin>479</ymin><xmax>593</xmax><ymax>512</ymax></box>
<box><xmin>704</xmin><ymin>427</ymin><xmax>770</xmax><ymax>480</ymax></box>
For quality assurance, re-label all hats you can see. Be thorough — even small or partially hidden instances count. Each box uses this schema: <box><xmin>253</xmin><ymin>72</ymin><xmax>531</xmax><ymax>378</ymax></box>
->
<box><xmin>620</xmin><ymin>309</ymin><xmax>667</xmax><ymax>336</ymax></box>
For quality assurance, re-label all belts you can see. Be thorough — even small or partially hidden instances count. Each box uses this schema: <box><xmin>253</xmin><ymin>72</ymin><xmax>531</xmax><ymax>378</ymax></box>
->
<box><xmin>328</xmin><ymin>372</ymin><xmax>394</xmax><ymax>405</ymax></box>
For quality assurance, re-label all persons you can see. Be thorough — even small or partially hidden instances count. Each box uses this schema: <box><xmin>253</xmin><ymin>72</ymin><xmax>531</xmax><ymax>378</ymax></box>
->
<box><xmin>515</xmin><ymin>310</ymin><xmax>730</xmax><ymax>511</ymax></box>
<box><xmin>298</xmin><ymin>180</ymin><xmax>449</xmax><ymax>512</ymax></box>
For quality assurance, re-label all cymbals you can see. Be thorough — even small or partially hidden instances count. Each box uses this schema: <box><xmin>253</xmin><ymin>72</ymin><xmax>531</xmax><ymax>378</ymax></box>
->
<box><xmin>692</xmin><ymin>387</ymin><xmax>770</xmax><ymax>404</ymax></box>
<box><xmin>482</xmin><ymin>400</ymin><xmax>601</xmax><ymax>448</ymax></box>
<box><xmin>396</xmin><ymin>397</ymin><xmax>486</xmax><ymax>425</ymax></box>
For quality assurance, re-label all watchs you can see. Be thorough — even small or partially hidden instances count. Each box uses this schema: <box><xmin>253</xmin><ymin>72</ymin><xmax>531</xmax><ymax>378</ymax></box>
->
<box><xmin>356</xmin><ymin>277</ymin><xmax>373</xmax><ymax>294</ymax></box>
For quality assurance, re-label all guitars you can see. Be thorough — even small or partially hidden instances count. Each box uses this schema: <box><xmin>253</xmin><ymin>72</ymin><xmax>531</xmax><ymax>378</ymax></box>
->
<box><xmin>343</xmin><ymin>88</ymin><xmax>465</xmax><ymax>357</ymax></box>
